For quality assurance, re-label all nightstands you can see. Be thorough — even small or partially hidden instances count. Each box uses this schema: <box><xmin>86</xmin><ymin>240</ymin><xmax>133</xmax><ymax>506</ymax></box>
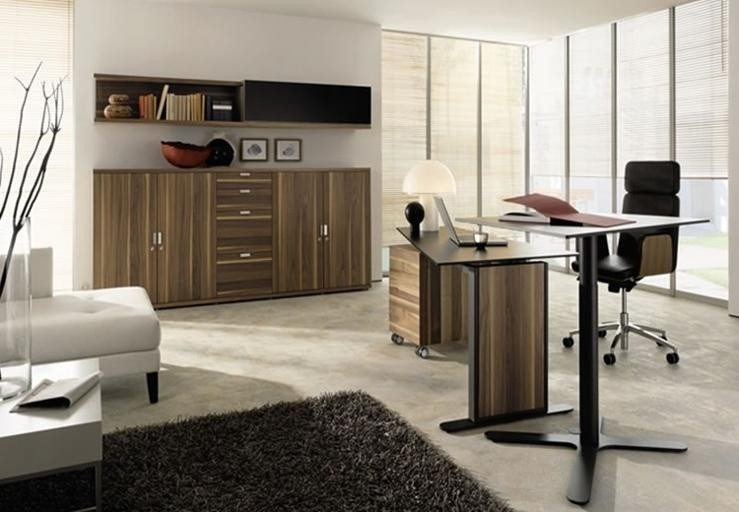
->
<box><xmin>0</xmin><ymin>357</ymin><xmax>105</xmax><ymax>512</ymax></box>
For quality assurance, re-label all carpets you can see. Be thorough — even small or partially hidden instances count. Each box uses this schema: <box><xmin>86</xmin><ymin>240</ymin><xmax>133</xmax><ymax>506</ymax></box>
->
<box><xmin>0</xmin><ymin>390</ymin><xmax>513</xmax><ymax>511</ymax></box>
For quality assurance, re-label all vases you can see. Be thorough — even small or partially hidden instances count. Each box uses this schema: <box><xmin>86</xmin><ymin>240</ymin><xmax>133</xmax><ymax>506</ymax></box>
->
<box><xmin>0</xmin><ymin>214</ymin><xmax>33</xmax><ymax>401</ymax></box>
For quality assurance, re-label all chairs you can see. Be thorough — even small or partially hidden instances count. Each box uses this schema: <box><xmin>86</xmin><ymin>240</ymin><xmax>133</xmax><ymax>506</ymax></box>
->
<box><xmin>562</xmin><ymin>162</ymin><xmax>680</xmax><ymax>366</ymax></box>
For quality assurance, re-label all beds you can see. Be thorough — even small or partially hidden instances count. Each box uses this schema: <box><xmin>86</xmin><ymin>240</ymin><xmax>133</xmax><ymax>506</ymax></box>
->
<box><xmin>0</xmin><ymin>244</ymin><xmax>163</xmax><ymax>403</ymax></box>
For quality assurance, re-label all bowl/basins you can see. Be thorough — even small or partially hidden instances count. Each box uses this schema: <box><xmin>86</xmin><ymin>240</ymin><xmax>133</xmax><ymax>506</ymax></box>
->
<box><xmin>159</xmin><ymin>138</ymin><xmax>213</xmax><ymax>169</ymax></box>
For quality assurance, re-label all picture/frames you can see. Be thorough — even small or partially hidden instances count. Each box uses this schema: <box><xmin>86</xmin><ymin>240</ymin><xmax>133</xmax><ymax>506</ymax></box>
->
<box><xmin>275</xmin><ymin>138</ymin><xmax>301</xmax><ymax>161</ymax></box>
<box><xmin>240</xmin><ymin>138</ymin><xmax>268</xmax><ymax>161</ymax></box>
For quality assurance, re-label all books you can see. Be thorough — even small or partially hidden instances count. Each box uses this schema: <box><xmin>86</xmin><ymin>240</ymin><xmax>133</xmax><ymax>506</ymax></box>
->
<box><xmin>497</xmin><ymin>192</ymin><xmax>638</xmax><ymax>228</ymax></box>
<box><xmin>135</xmin><ymin>84</ymin><xmax>236</xmax><ymax>123</ymax></box>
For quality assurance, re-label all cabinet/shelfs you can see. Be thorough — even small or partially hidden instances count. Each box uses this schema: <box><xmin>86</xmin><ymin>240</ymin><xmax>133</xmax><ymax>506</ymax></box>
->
<box><xmin>93</xmin><ymin>73</ymin><xmax>372</xmax><ymax>129</ymax></box>
<box><xmin>272</xmin><ymin>167</ymin><xmax>373</xmax><ymax>294</ymax></box>
<box><xmin>92</xmin><ymin>170</ymin><xmax>216</xmax><ymax>308</ymax></box>
<box><xmin>216</xmin><ymin>171</ymin><xmax>272</xmax><ymax>302</ymax></box>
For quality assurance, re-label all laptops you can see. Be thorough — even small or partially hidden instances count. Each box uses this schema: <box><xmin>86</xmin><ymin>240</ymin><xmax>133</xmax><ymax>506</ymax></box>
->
<box><xmin>434</xmin><ymin>196</ymin><xmax>507</xmax><ymax>246</ymax></box>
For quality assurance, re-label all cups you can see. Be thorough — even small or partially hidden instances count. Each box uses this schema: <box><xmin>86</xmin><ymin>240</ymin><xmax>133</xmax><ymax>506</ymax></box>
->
<box><xmin>1</xmin><ymin>213</ymin><xmax>37</xmax><ymax>404</ymax></box>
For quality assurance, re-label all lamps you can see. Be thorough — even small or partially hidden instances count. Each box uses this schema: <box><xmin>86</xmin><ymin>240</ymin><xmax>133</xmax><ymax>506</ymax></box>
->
<box><xmin>402</xmin><ymin>159</ymin><xmax>458</xmax><ymax>231</ymax></box>
